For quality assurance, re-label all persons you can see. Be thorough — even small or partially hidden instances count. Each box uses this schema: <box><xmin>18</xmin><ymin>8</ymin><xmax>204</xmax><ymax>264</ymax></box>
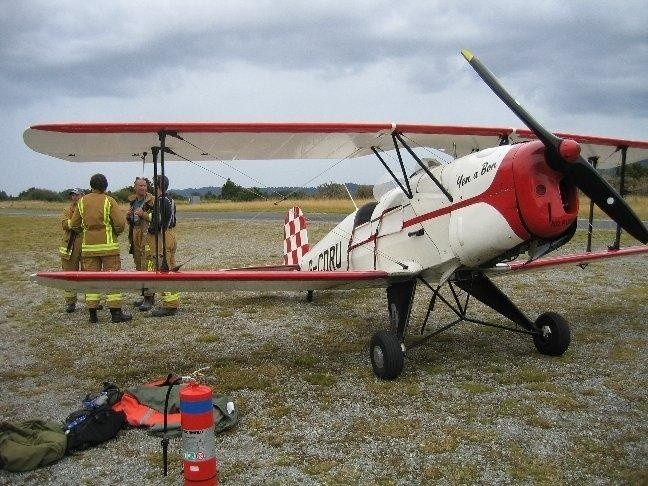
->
<box><xmin>146</xmin><ymin>175</ymin><xmax>181</xmax><ymax>316</ymax></box>
<box><xmin>125</xmin><ymin>179</ymin><xmax>155</xmax><ymax>311</ymax></box>
<box><xmin>70</xmin><ymin>174</ymin><xmax>132</xmax><ymax>322</ymax></box>
<box><xmin>58</xmin><ymin>188</ymin><xmax>103</xmax><ymax>312</ymax></box>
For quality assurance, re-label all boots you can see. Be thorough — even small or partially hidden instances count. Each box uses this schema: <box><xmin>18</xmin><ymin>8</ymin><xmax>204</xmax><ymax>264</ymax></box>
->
<box><xmin>88</xmin><ymin>308</ymin><xmax>98</xmax><ymax>322</ymax></box>
<box><xmin>135</xmin><ymin>298</ymin><xmax>142</xmax><ymax>306</ymax></box>
<box><xmin>140</xmin><ymin>295</ymin><xmax>153</xmax><ymax>309</ymax></box>
<box><xmin>110</xmin><ymin>308</ymin><xmax>133</xmax><ymax>323</ymax></box>
<box><xmin>66</xmin><ymin>303</ymin><xmax>74</xmax><ymax>312</ymax></box>
<box><xmin>154</xmin><ymin>307</ymin><xmax>175</xmax><ymax>317</ymax></box>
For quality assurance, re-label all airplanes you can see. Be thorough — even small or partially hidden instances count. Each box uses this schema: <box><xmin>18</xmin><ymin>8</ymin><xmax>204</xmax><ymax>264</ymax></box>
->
<box><xmin>24</xmin><ymin>48</ymin><xmax>648</xmax><ymax>381</ymax></box>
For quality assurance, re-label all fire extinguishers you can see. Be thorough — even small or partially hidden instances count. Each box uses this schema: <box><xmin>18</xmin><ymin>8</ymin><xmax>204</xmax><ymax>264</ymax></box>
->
<box><xmin>160</xmin><ymin>365</ymin><xmax>218</xmax><ymax>481</ymax></box>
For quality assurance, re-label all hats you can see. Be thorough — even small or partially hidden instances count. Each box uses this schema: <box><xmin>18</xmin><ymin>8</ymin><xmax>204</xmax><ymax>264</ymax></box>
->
<box><xmin>70</xmin><ymin>188</ymin><xmax>86</xmax><ymax>195</ymax></box>
